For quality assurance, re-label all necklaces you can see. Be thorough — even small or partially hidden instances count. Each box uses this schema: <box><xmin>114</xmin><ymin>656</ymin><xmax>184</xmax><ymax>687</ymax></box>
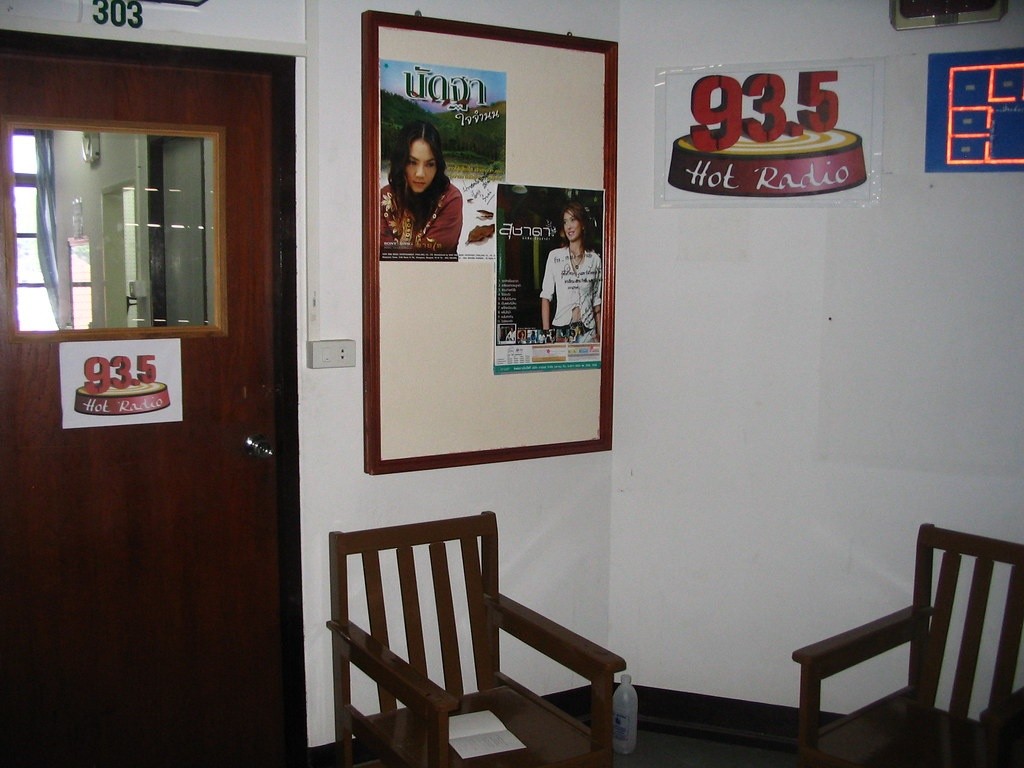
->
<box><xmin>574</xmin><ymin>259</ymin><xmax>581</xmax><ymax>269</ymax></box>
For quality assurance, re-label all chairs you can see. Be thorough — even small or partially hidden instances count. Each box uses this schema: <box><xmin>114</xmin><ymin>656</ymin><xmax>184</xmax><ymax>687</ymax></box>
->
<box><xmin>326</xmin><ymin>510</ymin><xmax>627</xmax><ymax>768</ymax></box>
<box><xmin>787</xmin><ymin>520</ymin><xmax>1024</xmax><ymax>768</ymax></box>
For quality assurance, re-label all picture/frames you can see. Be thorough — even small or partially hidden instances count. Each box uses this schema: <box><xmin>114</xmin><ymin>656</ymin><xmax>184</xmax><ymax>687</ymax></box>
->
<box><xmin>361</xmin><ymin>11</ymin><xmax>620</xmax><ymax>473</ymax></box>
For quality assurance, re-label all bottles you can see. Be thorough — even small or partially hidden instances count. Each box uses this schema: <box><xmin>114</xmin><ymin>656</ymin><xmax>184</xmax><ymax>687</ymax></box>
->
<box><xmin>612</xmin><ymin>673</ymin><xmax>639</xmax><ymax>754</ymax></box>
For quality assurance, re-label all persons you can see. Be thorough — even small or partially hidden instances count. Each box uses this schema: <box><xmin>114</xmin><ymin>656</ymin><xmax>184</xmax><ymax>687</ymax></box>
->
<box><xmin>539</xmin><ymin>203</ymin><xmax>602</xmax><ymax>342</ymax></box>
<box><xmin>380</xmin><ymin>121</ymin><xmax>463</xmax><ymax>261</ymax></box>
<box><xmin>505</xmin><ymin>327</ymin><xmax>576</xmax><ymax>344</ymax></box>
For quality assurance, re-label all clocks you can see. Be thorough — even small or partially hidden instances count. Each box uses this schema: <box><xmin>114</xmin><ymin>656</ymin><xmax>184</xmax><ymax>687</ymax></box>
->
<box><xmin>82</xmin><ymin>132</ymin><xmax>100</xmax><ymax>163</ymax></box>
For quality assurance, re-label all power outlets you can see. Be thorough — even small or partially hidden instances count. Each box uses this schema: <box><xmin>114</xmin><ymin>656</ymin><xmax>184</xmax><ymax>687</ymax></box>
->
<box><xmin>306</xmin><ymin>339</ymin><xmax>356</xmax><ymax>369</ymax></box>
